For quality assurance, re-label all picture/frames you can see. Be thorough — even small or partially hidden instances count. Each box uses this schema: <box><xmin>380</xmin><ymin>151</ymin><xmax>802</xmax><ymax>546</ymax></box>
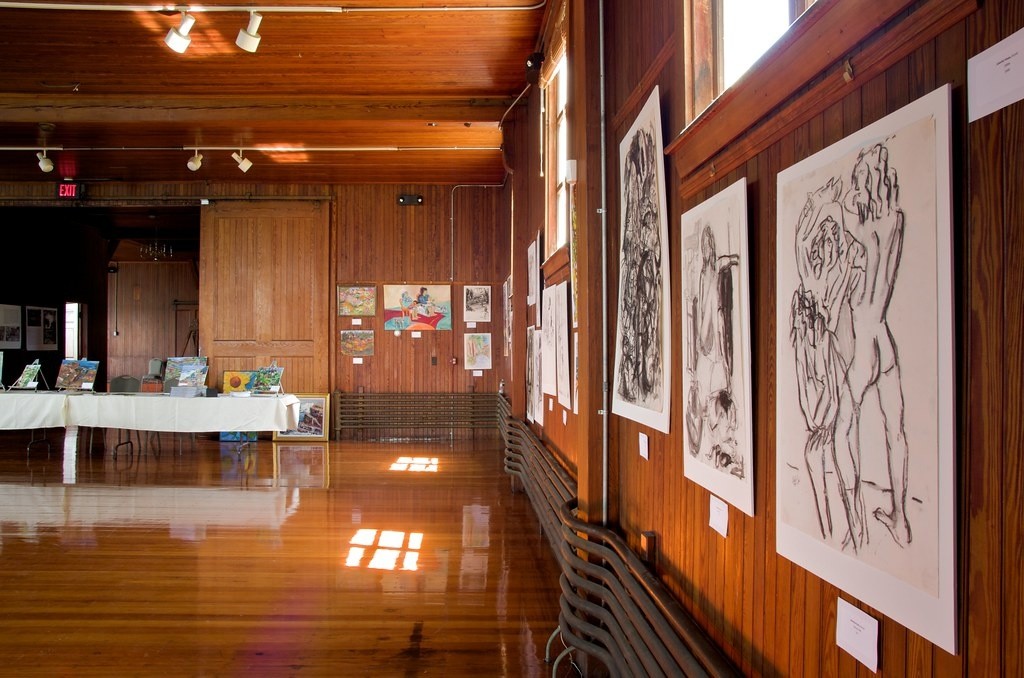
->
<box><xmin>272</xmin><ymin>393</ymin><xmax>330</xmax><ymax>442</ymax></box>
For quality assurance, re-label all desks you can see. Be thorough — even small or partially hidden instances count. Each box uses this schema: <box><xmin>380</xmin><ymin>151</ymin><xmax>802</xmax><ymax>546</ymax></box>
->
<box><xmin>0</xmin><ymin>390</ymin><xmax>301</xmax><ymax>461</ymax></box>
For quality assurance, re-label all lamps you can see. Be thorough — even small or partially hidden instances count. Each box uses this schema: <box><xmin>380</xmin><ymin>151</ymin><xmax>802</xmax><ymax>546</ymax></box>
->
<box><xmin>36</xmin><ymin>150</ymin><xmax>54</xmax><ymax>173</ymax></box>
<box><xmin>187</xmin><ymin>150</ymin><xmax>203</xmax><ymax>171</ymax></box>
<box><xmin>138</xmin><ymin>239</ymin><xmax>172</xmax><ymax>261</ymax></box>
<box><xmin>164</xmin><ymin>12</ymin><xmax>195</xmax><ymax>54</ymax></box>
<box><xmin>235</xmin><ymin>11</ymin><xmax>263</xmax><ymax>53</ymax></box>
<box><xmin>231</xmin><ymin>151</ymin><xmax>253</xmax><ymax>173</ymax></box>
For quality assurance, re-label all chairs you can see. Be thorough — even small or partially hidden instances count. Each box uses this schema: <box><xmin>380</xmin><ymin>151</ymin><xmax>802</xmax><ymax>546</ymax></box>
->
<box><xmin>110</xmin><ymin>375</ymin><xmax>207</xmax><ymax>457</ymax></box>
<box><xmin>141</xmin><ymin>358</ymin><xmax>164</xmax><ymax>392</ymax></box>
